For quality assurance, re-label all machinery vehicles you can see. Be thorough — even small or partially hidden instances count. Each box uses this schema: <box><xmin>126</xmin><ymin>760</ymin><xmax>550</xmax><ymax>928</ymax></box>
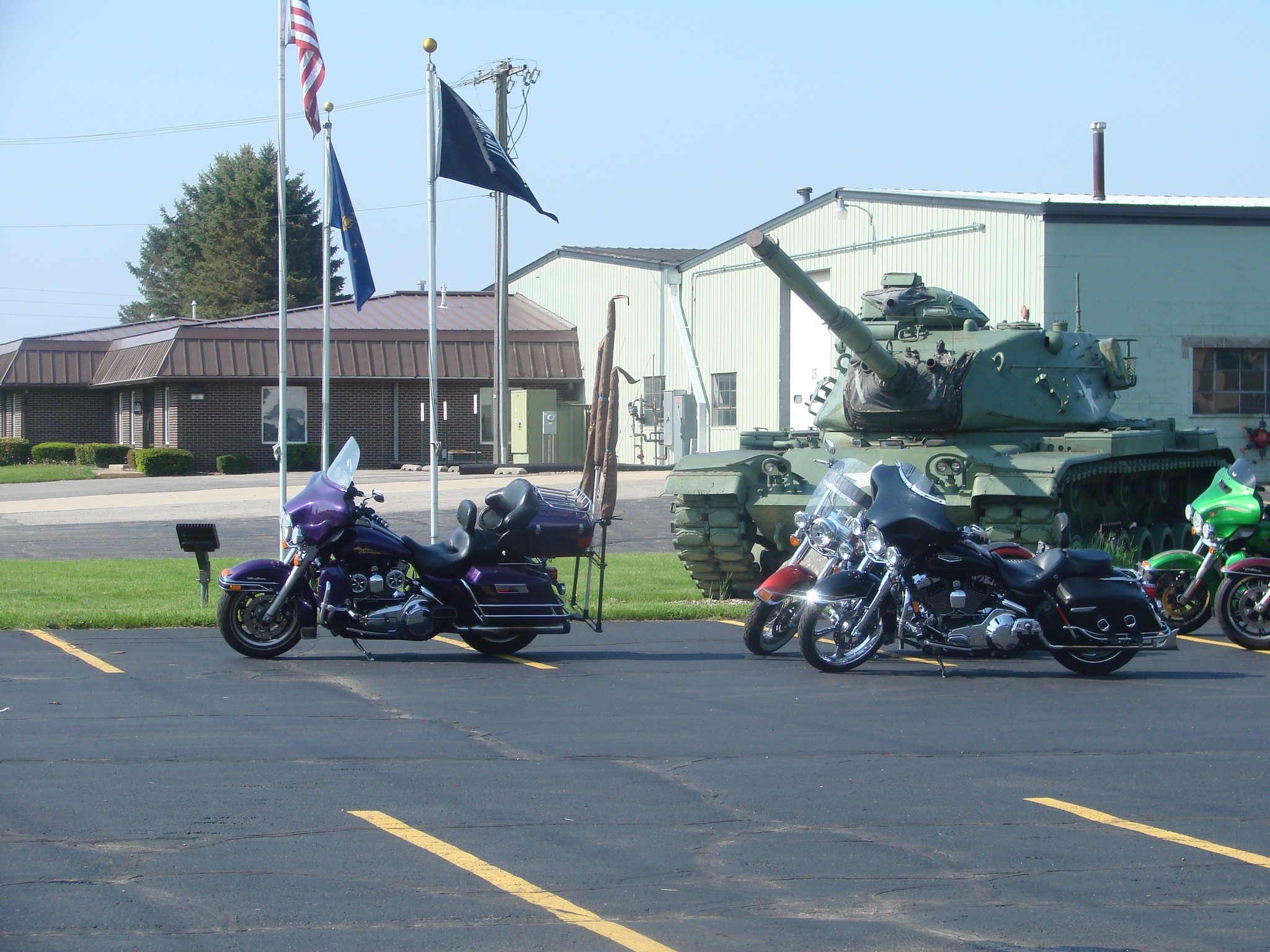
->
<box><xmin>663</xmin><ymin>228</ymin><xmax>1247</xmax><ymax>603</ymax></box>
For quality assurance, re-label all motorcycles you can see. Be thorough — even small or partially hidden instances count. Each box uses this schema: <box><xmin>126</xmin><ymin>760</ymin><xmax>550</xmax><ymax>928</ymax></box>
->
<box><xmin>1214</xmin><ymin>556</ymin><xmax>1270</xmax><ymax>652</ymax></box>
<box><xmin>1133</xmin><ymin>456</ymin><xmax>1270</xmax><ymax>636</ymax></box>
<box><xmin>743</xmin><ymin>438</ymin><xmax>1178</xmax><ymax>675</ymax></box>
<box><xmin>214</xmin><ymin>433</ymin><xmax>611</xmax><ymax>660</ymax></box>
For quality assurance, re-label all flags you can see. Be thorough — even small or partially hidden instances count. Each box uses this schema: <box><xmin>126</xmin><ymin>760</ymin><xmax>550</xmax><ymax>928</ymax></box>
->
<box><xmin>427</xmin><ymin>74</ymin><xmax>558</xmax><ymax>219</ymax></box>
<box><xmin>279</xmin><ymin>0</ymin><xmax>325</xmax><ymax>134</ymax></box>
<box><xmin>324</xmin><ymin>126</ymin><xmax>376</xmax><ymax>312</ymax></box>
